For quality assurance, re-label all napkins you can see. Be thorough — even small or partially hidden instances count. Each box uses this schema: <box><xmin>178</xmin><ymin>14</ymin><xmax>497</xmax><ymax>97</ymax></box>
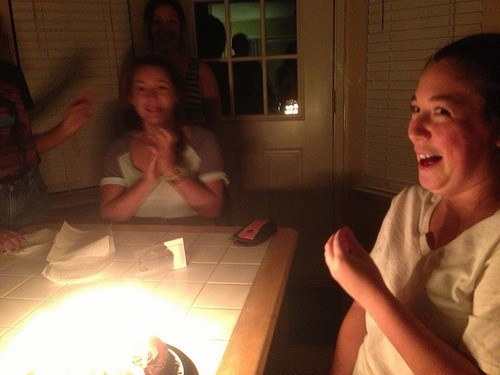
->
<box><xmin>4</xmin><ymin>228</ymin><xmax>57</xmax><ymax>256</ymax></box>
<box><xmin>42</xmin><ymin>222</ymin><xmax>116</xmax><ymax>286</ymax></box>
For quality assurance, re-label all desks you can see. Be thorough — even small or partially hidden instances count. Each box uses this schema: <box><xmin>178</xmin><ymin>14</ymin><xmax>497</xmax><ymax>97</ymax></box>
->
<box><xmin>0</xmin><ymin>223</ymin><xmax>299</xmax><ymax>375</ymax></box>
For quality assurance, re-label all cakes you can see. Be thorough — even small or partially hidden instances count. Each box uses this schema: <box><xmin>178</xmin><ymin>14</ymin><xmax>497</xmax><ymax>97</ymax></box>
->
<box><xmin>133</xmin><ymin>336</ymin><xmax>198</xmax><ymax>375</ymax></box>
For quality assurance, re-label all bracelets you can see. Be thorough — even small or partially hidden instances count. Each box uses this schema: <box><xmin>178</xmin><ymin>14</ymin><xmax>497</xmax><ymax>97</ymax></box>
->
<box><xmin>164</xmin><ymin>165</ymin><xmax>189</xmax><ymax>185</ymax></box>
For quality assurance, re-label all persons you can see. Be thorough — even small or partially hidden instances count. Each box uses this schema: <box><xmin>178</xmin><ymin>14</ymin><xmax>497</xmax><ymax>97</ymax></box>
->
<box><xmin>276</xmin><ymin>42</ymin><xmax>298</xmax><ymax>103</ymax></box>
<box><xmin>142</xmin><ymin>0</ymin><xmax>222</xmax><ymax>139</ymax></box>
<box><xmin>225</xmin><ymin>31</ymin><xmax>278</xmax><ymax>115</ymax></box>
<box><xmin>322</xmin><ymin>30</ymin><xmax>500</xmax><ymax>375</ymax></box>
<box><xmin>0</xmin><ymin>61</ymin><xmax>94</xmax><ymax>254</ymax></box>
<box><xmin>96</xmin><ymin>41</ymin><xmax>231</xmax><ymax>225</ymax></box>
